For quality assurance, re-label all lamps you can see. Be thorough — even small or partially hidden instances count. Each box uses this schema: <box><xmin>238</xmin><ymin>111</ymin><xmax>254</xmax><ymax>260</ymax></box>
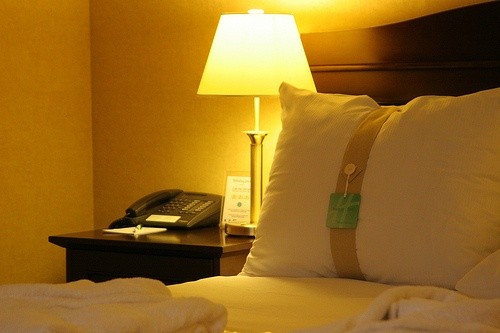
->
<box><xmin>194</xmin><ymin>7</ymin><xmax>319</xmax><ymax>237</ymax></box>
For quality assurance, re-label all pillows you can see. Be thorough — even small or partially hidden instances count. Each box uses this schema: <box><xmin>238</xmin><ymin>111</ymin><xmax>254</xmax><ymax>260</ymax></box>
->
<box><xmin>242</xmin><ymin>80</ymin><xmax>499</xmax><ymax>288</ymax></box>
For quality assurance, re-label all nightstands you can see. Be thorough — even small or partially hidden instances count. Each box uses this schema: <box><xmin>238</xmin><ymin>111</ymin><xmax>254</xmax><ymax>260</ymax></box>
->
<box><xmin>47</xmin><ymin>228</ymin><xmax>254</xmax><ymax>287</ymax></box>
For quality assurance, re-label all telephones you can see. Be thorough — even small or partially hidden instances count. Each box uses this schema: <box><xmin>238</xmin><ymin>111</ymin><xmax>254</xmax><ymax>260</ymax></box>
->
<box><xmin>106</xmin><ymin>188</ymin><xmax>224</xmax><ymax>231</ymax></box>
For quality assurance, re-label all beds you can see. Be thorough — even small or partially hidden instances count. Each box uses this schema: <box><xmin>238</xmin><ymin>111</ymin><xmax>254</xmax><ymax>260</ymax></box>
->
<box><xmin>0</xmin><ymin>263</ymin><xmax>499</xmax><ymax>332</ymax></box>
